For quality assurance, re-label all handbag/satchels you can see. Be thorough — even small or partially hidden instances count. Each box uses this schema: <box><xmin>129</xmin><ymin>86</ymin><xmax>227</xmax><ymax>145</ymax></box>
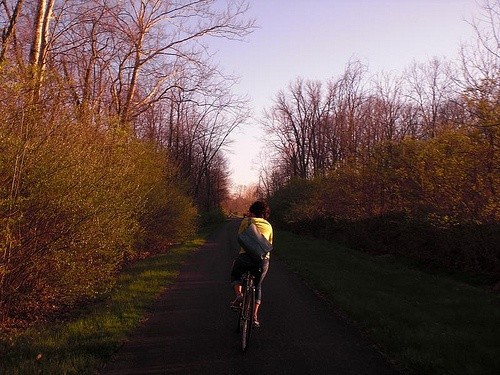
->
<box><xmin>237</xmin><ymin>223</ymin><xmax>273</xmax><ymax>268</ymax></box>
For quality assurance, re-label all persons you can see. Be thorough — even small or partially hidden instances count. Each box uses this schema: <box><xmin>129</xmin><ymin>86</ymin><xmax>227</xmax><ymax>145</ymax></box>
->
<box><xmin>230</xmin><ymin>200</ymin><xmax>274</xmax><ymax>328</ymax></box>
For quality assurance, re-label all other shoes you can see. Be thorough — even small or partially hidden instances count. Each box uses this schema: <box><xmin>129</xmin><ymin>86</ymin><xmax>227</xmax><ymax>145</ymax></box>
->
<box><xmin>251</xmin><ymin>321</ymin><xmax>260</xmax><ymax>329</ymax></box>
<box><xmin>231</xmin><ymin>293</ymin><xmax>244</xmax><ymax>307</ymax></box>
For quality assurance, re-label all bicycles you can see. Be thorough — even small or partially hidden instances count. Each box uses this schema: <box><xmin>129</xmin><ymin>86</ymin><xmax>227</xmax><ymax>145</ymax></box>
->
<box><xmin>230</xmin><ymin>266</ymin><xmax>262</xmax><ymax>354</ymax></box>
